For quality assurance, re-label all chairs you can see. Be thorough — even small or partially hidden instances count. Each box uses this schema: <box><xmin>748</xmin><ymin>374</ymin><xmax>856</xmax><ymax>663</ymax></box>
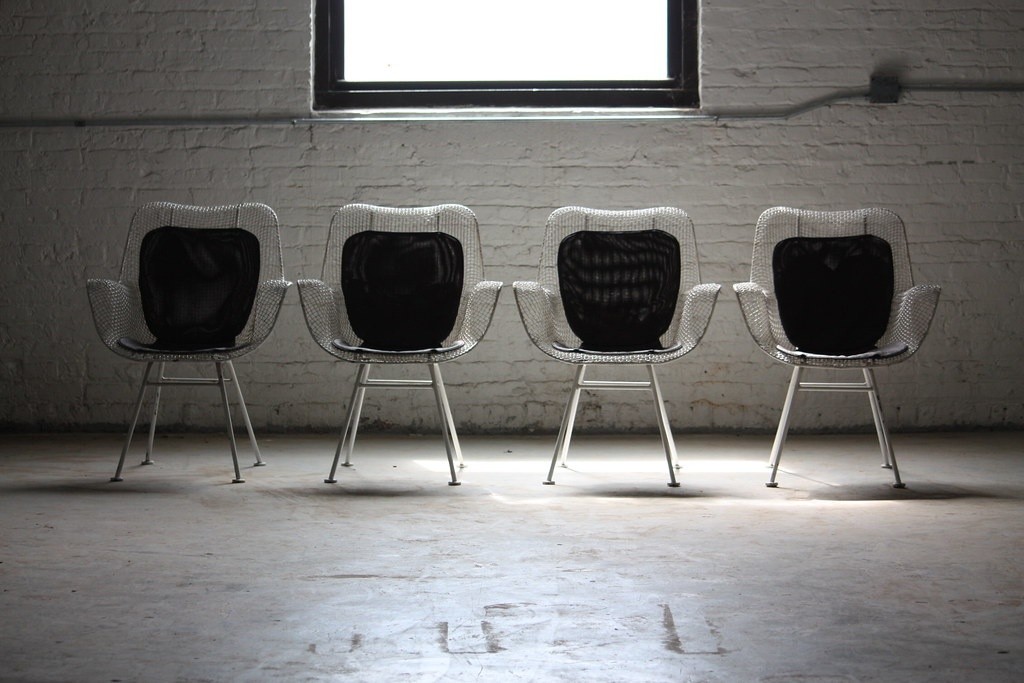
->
<box><xmin>515</xmin><ymin>206</ymin><xmax>723</xmax><ymax>488</ymax></box>
<box><xmin>296</xmin><ymin>204</ymin><xmax>505</xmax><ymax>487</ymax></box>
<box><xmin>86</xmin><ymin>202</ymin><xmax>292</xmax><ymax>483</ymax></box>
<box><xmin>732</xmin><ymin>206</ymin><xmax>942</xmax><ymax>489</ymax></box>
